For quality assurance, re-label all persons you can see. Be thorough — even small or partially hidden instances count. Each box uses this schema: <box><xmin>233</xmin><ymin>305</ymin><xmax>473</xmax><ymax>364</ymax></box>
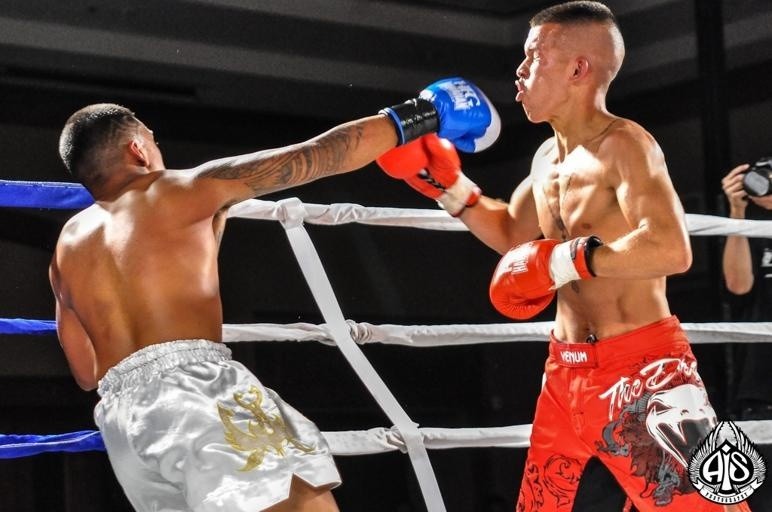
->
<box><xmin>49</xmin><ymin>75</ymin><xmax>504</xmax><ymax>511</ymax></box>
<box><xmin>721</xmin><ymin>154</ymin><xmax>772</xmax><ymax>421</ymax></box>
<box><xmin>376</xmin><ymin>0</ymin><xmax>750</xmax><ymax>511</ymax></box>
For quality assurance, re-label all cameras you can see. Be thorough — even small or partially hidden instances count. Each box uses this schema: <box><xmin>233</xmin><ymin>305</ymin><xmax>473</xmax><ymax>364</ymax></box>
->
<box><xmin>743</xmin><ymin>159</ymin><xmax>772</xmax><ymax>199</ymax></box>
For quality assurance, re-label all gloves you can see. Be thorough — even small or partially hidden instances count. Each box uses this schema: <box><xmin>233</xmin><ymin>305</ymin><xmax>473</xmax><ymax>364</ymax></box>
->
<box><xmin>490</xmin><ymin>235</ymin><xmax>600</xmax><ymax>319</ymax></box>
<box><xmin>377</xmin><ymin>133</ymin><xmax>480</xmax><ymax>215</ymax></box>
<box><xmin>379</xmin><ymin>79</ymin><xmax>502</xmax><ymax>154</ymax></box>
